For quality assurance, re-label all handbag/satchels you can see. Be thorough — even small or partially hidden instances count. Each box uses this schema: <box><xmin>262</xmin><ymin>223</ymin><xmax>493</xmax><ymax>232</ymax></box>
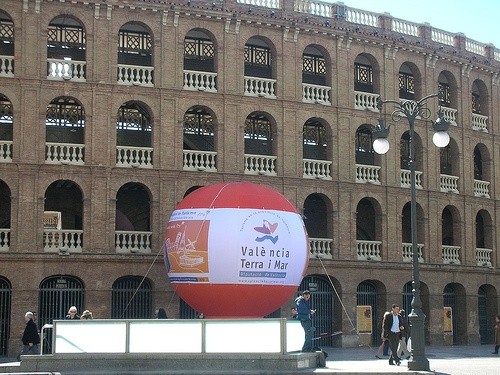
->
<box><xmin>407</xmin><ymin>337</ymin><xmax>412</xmax><ymax>353</ymax></box>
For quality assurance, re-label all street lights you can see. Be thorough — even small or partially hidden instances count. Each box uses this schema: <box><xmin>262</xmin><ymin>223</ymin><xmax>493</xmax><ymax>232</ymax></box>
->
<box><xmin>374</xmin><ymin>91</ymin><xmax>459</xmax><ymax>373</ymax></box>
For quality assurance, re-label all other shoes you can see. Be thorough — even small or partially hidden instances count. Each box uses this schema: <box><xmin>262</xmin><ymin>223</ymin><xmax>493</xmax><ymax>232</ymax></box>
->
<box><xmin>492</xmin><ymin>352</ymin><xmax>498</xmax><ymax>354</ymax></box>
<box><xmin>406</xmin><ymin>356</ymin><xmax>411</xmax><ymax>360</ymax></box>
<box><xmin>397</xmin><ymin>362</ymin><xmax>401</xmax><ymax>366</ymax></box>
<box><xmin>389</xmin><ymin>363</ymin><xmax>395</xmax><ymax>365</ymax></box>
<box><xmin>376</xmin><ymin>355</ymin><xmax>382</xmax><ymax>359</ymax></box>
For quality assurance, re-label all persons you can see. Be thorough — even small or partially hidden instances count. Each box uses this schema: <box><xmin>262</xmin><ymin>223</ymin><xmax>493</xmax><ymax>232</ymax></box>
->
<box><xmin>492</xmin><ymin>312</ymin><xmax>500</xmax><ymax>354</ymax></box>
<box><xmin>376</xmin><ymin>304</ymin><xmax>411</xmax><ymax>366</ymax></box>
<box><xmin>66</xmin><ymin>306</ymin><xmax>79</xmax><ymax>319</ymax></box>
<box><xmin>196</xmin><ymin>312</ymin><xmax>204</xmax><ymax>319</ymax></box>
<box><xmin>17</xmin><ymin>312</ymin><xmax>41</xmax><ymax>362</ymax></box>
<box><xmin>80</xmin><ymin>310</ymin><xmax>93</xmax><ymax>319</ymax></box>
<box><xmin>291</xmin><ymin>290</ymin><xmax>316</xmax><ymax>352</ymax></box>
<box><xmin>155</xmin><ymin>308</ymin><xmax>169</xmax><ymax>319</ymax></box>
